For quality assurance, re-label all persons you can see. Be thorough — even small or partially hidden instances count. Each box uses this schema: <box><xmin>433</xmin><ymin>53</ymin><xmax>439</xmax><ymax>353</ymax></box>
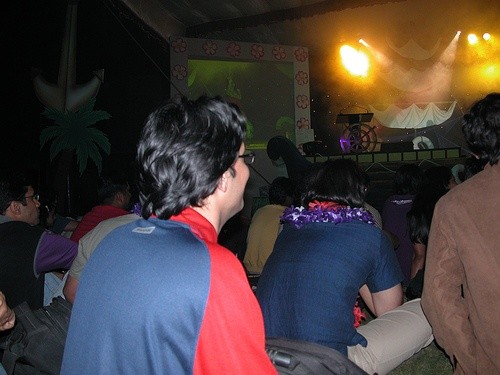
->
<box><xmin>218</xmin><ymin>133</ymin><xmax>489</xmax><ymax>301</ymax></box>
<box><xmin>56</xmin><ymin>96</ymin><xmax>279</xmax><ymax>375</ymax></box>
<box><xmin>253</xmin><ymin>159</ymin><xmax>436</xmax><ymax>375</ymax></box>
<box><xmin>420</xmin><ymin>93</ymin><xmax>500</xmax><ymax>375</ymax></box>
<box><xmin>0</xmin><ymin>146</ymin><xmax>159</xmax><ymax>375</ymax></box>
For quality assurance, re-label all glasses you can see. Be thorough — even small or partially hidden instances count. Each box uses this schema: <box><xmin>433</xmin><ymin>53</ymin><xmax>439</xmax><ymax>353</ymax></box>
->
<box><xmin>24</xmin><ymin>194</ymin><xmax>39</xmax><ymax>201</ymax></box>
<box><xmin>238</xmin><ymin>149</ymin><xmax>256</xmax><ymax>164</ymax></box>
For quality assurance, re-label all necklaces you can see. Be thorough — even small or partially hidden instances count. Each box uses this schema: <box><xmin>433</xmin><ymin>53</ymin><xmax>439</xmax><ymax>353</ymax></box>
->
<box><xmin>279</xmin><ymin>200</ymin><xmax>376</xmax><ymax>330</ymax></box>
<box><xmin>131</xmin><ymin>202</ymin><xmax>142</xmax><ymax>217</ymax></box>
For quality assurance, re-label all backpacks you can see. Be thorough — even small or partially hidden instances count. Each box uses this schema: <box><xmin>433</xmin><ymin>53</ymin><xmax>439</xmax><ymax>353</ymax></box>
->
<box><xmin>263</xmin><ymin>336</ymin><xmax>367</xmax><ymax>375</ymax></box>
<box><xmin>1</xmin><ymin>296</ymin><xmax>74</xmax><ymax>375</ymax></box>
